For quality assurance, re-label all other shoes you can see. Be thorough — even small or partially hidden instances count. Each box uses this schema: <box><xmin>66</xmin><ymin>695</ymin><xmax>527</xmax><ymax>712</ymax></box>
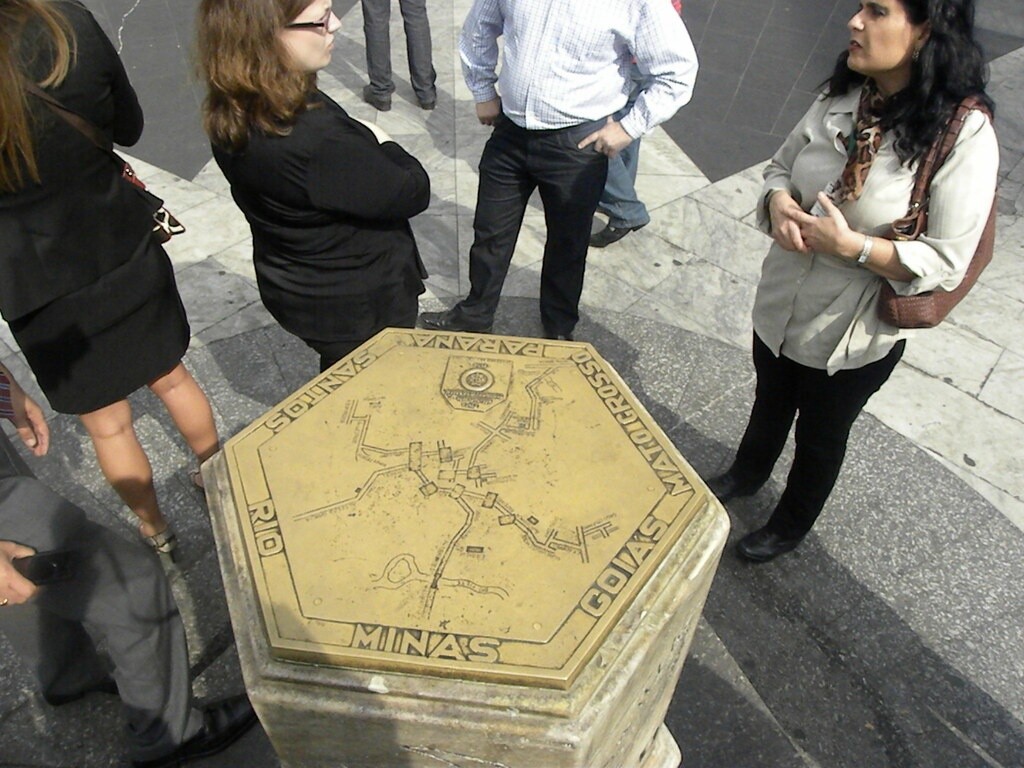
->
<box><xmin>590</xmin><ymin>210</ymin><xmax>650</xmax><ymax>249</ymax></box>
<box><xmin>364</xmin><ymin>84</ymin><xmax>391</xmax><ymax>111</ymax></box>
<box><xmin>416</xmin><ymin>80</ymin><xmax>436</xmax><ymax>109</ymax></box>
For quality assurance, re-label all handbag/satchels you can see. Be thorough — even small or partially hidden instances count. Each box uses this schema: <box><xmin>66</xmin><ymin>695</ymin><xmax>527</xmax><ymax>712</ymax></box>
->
<box><xmin>885</xmin><ymin>186</ymin><xmax>1001</xmax><ymax>328</ymax></box>
<box><xmin>122</xmin><ymin>163</ymin><xmax>187</xmax><ymax>245</ymax></box>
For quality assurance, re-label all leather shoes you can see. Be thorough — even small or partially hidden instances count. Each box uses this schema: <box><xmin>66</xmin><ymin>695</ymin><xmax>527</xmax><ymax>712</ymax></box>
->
<box><xmin>705</xmin><ymin>469</ymin><xmax>759</xmax><ymax>502</ymax></box>
<box><xmin>419</xmin><ymin>307</ymin><xmax>495</xmax><ymax>334</ymax></box>
<box><xmin>130</xmin><ymin>692</ymin><xmax>258</xmax><ymax>767</ymax></box>
<box><xmin>736</xmin><ymin>526</ymin><xmax>799</xmax><ymax>562</ymax></box>
<box><xmin>550</xmin><ymin>329</ymin><xmax>572</xmax><ymax>340</ymax></box>
<box><xmin>42</xmin><ymin>678</ymin><xmax>119</xmax><ymax>706</ymax></box>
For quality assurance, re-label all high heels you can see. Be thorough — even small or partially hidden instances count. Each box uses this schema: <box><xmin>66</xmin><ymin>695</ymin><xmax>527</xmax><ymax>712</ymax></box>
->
<box><xmin>187</xmin><ymin>468</ymin><xmax>208</xmax><ymax>492</ymax></box>
<box><xmin>138</xmin><ymin>530</ymin><xmax>181</xmax><ymax>564</ymax></box>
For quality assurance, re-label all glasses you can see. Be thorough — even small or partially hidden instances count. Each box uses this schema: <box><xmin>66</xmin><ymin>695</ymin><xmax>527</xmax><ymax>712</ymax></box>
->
<box><xmin>274</xmin><ymin>15</ymin><xmax>334</xmax><ymax>34</ymax></box>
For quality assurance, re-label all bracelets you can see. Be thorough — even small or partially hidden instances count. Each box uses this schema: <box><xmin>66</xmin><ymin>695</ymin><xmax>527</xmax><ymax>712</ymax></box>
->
<box><xmin>858</xmin><ymin>234</ymin><xmax>872</xmax><ymax>265</ymax></box>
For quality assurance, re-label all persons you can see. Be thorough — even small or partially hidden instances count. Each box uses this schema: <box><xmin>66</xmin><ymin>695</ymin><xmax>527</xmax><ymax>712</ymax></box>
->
<box><xmin>197</xmin><ymin>0</ymin><xmax>431</xmax><ymax>374</ymax></box>
<box><xmin>0</xmin><ymin>361</ymin><xmax>257</xmax><ymax>768</ymax></box>
<box><xmin>423</xmin><ymin>0</ymin><xmax>699</xmax><ymax>342</ymax></box>
<box><xmin>706</xmin><ymin>0</ymin><xmax>1001</xmax><ymax>562</ymax></box>
<box><xmin>361</xmin><ymin>0</ymin><xmax>437</xmax><ymax>111</ymax></box>
<box><xmin>0</xmin><ymin>0</ymin><xmax>219</xmax><ymax>564</ymax></box>
<box><xmin>590</xmin><ymin>0</ymin><xmax>683</xmax><ymax>248</ymax></box>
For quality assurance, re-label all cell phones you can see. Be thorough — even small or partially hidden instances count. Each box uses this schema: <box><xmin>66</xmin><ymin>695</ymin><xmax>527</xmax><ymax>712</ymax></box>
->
<box><xmin>12</xmin><ymin>550</ymin><xmax>76</xmax><ymax>586</ymax></box>
